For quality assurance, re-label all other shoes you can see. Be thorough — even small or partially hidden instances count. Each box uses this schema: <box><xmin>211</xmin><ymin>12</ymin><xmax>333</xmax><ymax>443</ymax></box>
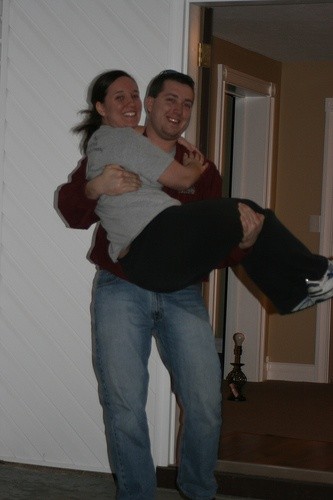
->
<box><xmin>291</xmin><ymin>295</ymin><xmax>323</xmax><ymax>312</ymax></box>
<box><xmin>305</xmin><ymin>256</ymin><xmax>332</xmax><ymax>297</ymax></box>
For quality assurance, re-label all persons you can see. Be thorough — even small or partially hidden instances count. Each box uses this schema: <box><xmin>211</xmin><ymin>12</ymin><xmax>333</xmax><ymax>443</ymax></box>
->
<box><xmin>54</xmin><ymin>68</ymin><xmax>265</xmax><ymax>500</ymax></box>
<box><xmin>69</xmin><ymin>71</ymin><xmax>333</xmax><ymax>315</ymax></box>
